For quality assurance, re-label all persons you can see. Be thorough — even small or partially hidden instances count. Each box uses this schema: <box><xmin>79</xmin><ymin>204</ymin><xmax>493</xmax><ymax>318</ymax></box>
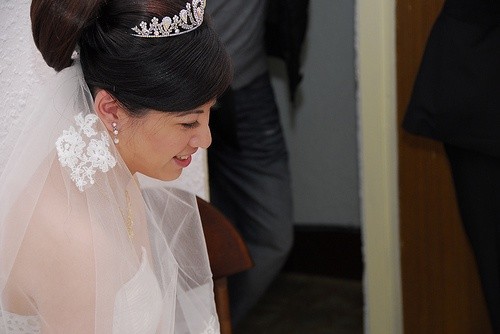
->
<box><xmin>1</xmin><ymin>0</ymin><xmax>234</xmax><ymax>334</ymax></box>
<box><xmin>205</xmin><ymin>1</ymin><xmax>310</xmax><ymax>334</ymax></box>
<box><xmin>400</xmin><ymin>0</ymin><xmax>500</xmax><ymax>334</ymax></box>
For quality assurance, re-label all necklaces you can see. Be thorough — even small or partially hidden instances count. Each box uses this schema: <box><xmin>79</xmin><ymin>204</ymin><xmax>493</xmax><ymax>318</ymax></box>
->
<box><xmin>87</xmin><ymin>165</ymin><xmax>135</xmax><ymax>243</ymax></box>
